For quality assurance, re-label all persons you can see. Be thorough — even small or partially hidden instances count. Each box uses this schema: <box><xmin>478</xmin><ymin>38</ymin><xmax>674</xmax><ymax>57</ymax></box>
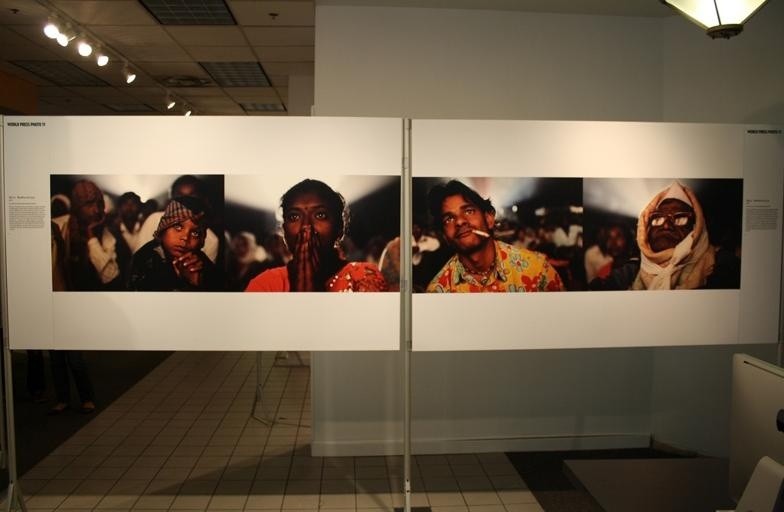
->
<box><xmin>52</xmin><ymin>176</ymin><xmax>401</xmax><ymax>292</ymax></box>
<box><xmin>411</xmin><ymin>180</ymin><xmax>740</xmax><ymax>292</ymax></box>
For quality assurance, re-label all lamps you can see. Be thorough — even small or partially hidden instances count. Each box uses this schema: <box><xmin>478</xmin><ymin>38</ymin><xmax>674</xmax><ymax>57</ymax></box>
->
<box><xmin>35</xmin><ymin>0</ymin><xmax>201</xmax><ymax>115</ymax></box>
<box><xmin>659</xmin><ymin>0</ymin><xmax>769</xmax><ymax>39</ymax></box>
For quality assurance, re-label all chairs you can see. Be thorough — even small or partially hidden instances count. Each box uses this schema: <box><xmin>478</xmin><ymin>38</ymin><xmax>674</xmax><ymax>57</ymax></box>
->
<box><xmin>735</xmin><ymin>455</ymin><xmax>783</xmax><ymax>511</ymax></box>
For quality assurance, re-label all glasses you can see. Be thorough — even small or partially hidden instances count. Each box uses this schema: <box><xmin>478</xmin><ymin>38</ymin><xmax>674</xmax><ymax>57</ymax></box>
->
<box><xmin>649</xmin><ymin>211</ymin><xmax>692</xmax><ymax>229</ymax></box>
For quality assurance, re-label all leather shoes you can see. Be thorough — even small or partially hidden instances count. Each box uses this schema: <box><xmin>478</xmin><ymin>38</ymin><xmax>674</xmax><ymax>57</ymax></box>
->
<box><xmin>46</xmin><ymin>401</ymin><xmax>97</xmax><ymax>414</ymax></box>
